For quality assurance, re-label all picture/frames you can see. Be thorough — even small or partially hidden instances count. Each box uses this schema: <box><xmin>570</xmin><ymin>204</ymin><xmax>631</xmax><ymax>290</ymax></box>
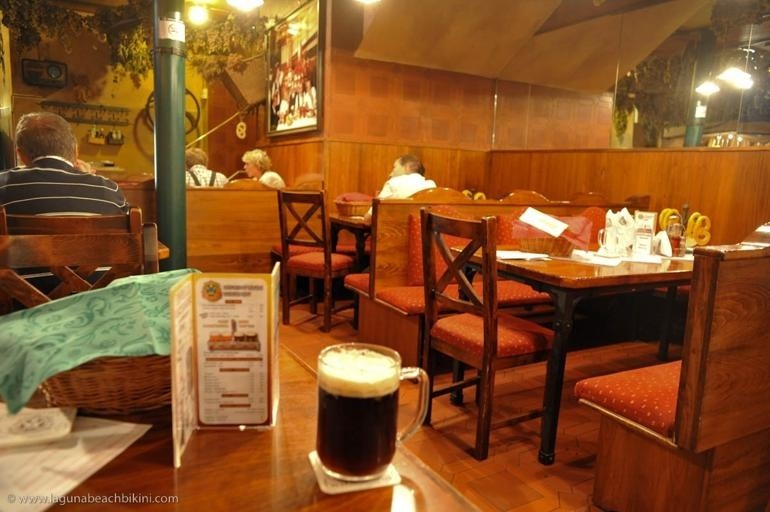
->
<box><xmin>263</xmin><ymin>1</ymin><xmax>323</xmax><ymax>139</ymax></box>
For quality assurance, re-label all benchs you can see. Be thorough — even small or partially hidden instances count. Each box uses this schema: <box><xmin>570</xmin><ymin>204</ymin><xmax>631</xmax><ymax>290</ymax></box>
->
<box><xmin>345</xmin><ymin>187</ymin><xmax>770</xmax><ymax>512</ymax></box>
<box><xmin>271</xmin><ymin>241</ymin><xmax>371</xmax><ymax>304</ymax></box>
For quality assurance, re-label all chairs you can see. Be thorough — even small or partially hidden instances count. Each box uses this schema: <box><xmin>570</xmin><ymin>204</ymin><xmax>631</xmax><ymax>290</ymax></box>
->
<box><xmin>0</xmin><ymin>207</ymin><xmax>161</xmax><ymax>317</ymax></box>
<box><xmin>275</xmin><ymin>188</ymin><xmax>359</xmax><ymax>333</ymax></box>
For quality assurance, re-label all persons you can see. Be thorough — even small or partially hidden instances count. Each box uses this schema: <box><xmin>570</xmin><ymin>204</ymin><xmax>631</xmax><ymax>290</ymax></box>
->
<box><xmin>185</xmin><ymin>147</ymin><xmax>228</xmax><ymax>191</ymax></box>
<box><xmin>2</xmin><ymin>112</ymin><xmax>129</xmax><ymax>314</ymax></box>
<box><xmin>363</xmin><ymin>155</ymin><xmax>436</xmax><ymax>269</ymax></box>
<box><xmin>243</xmin><ymin>148</ymin><xmax>287</xmax><ymax>189</ymax></box>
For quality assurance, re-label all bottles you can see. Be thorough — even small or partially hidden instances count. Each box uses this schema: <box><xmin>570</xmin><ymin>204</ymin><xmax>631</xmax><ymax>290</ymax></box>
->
<box><xmin>665</xmin><ymin>214</ymin><xmax>686</xmax><ymax>257</ymax></box>
<box><xmin>633</xmin><ymin>225</ymin><xmax>654</xmax><ymax>260</ymax></box>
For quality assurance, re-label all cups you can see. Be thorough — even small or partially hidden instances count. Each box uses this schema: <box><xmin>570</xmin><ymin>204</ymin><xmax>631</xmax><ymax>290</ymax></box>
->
<box><xmin>707</xmin><ymin>133</ymin><xmax>770</xmax><ymax>148</ymax></box>
<box><xmin>598</xmin><ymin>226</ymin><xmax>621</xmax><ymax>257</ymax></box>
<box><xmin>315</xmin><ymin>341</ymin><xmax>430</xmax><ymax>483</ymax></box>
